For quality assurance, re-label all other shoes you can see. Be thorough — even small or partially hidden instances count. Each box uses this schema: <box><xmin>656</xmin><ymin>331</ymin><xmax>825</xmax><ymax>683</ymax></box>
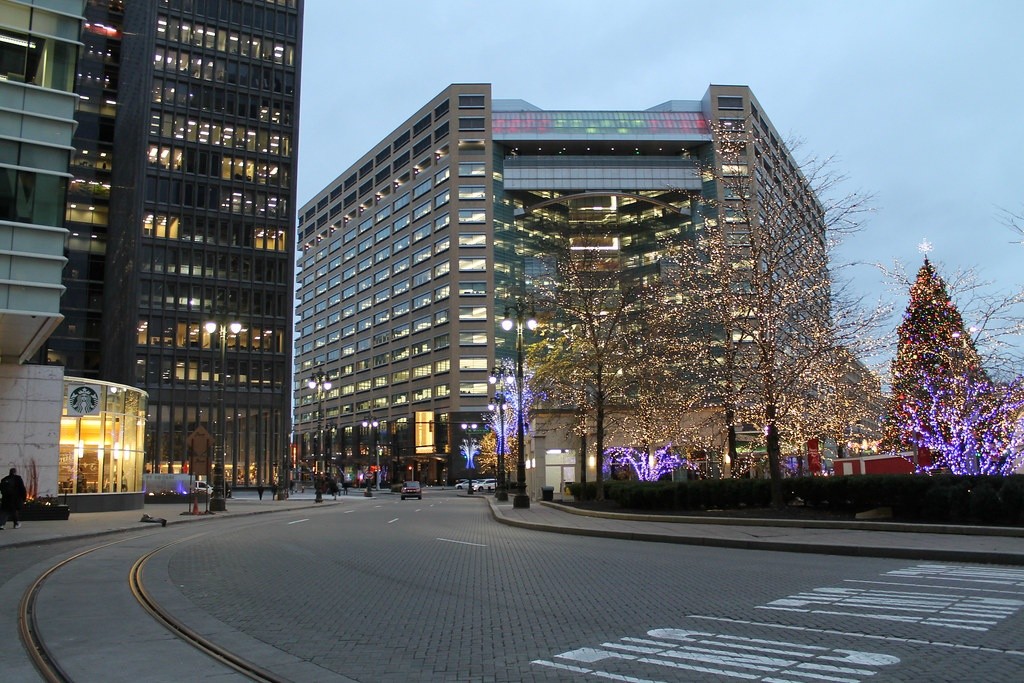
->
<box><xmin>0</xmin><ymin>525</ymin><xmax>6</xmax><ymax>530</ymax></box>
<box><xmin>14</xmin><ymin>523</ymin><xmax>21</xmax><ymax>528</ymax></box>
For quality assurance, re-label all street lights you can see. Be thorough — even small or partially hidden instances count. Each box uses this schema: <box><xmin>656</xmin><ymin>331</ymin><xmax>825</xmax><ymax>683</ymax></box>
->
<box><xmin>488</xmin><ymin>364</ymin><xmax>514</xmax><ymax>502</ymax></box>
<box><xmin>362</xmin><ymin>412</ymin><xmax>378</xmax><ymax>498</ymax></box>
<box><xmin>461</xmin><ymin>414</ymin><xmax>477</xmax><ymax>495</ymax></box>
<box><xmin>202</xmin><ymin>292</ymin><xmax>241</xmax><ymax>512</ymax></box>
<box><xmin>308</xmin><ymin>367</ymin><xmax>332</xmax><ymax>503</ymax></box>
<box><xmin>501</xmin><ymin>297</ymin><xmax>537</xmax><ymax>510</ymax></box>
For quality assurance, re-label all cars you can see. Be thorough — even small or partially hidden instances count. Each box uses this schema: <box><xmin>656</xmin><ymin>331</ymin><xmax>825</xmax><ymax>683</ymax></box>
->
<box><xmin>401</xmin><ymin>481</ymin><xmax>422</xmax><ymax>500</ymax></box>
<box><xmin>195</xmin><ymin>481</ymin><xmax>214</xmax><ymax>498</ymax></box>
<box><xmin>455</xmin><ymin>479</ymin><xmax>498</xmax><ymax>491</ymax></box>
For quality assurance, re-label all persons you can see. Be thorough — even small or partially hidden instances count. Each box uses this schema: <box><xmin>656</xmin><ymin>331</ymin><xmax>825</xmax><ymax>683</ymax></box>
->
<box><xmin>258</xmin><ymin>484</ymin><xmax>264</xmax><ymax>501</ymax></box>
<box><xmin>0</xmin><ymin>467</ymin><xmax>27</xmax><ymax>531</ymax></box>
<box><xmin>77</xmin><ymin>467</ymin><xmax>85</xmax><ymax>493</ymax></box>
<box><xmin>271</xmin><ymin>482</ymin><xmax>277</xmax><ymax>500</ymax></box>
<box><xmin>342</xmin><ymin>482</ymin><xmax>348</xmax><ymax>495</ymax></box>
<box><xmin>337</xmin><ymin>481</ymin><xmax>342</xmax><ymax>496</ymax></box>
